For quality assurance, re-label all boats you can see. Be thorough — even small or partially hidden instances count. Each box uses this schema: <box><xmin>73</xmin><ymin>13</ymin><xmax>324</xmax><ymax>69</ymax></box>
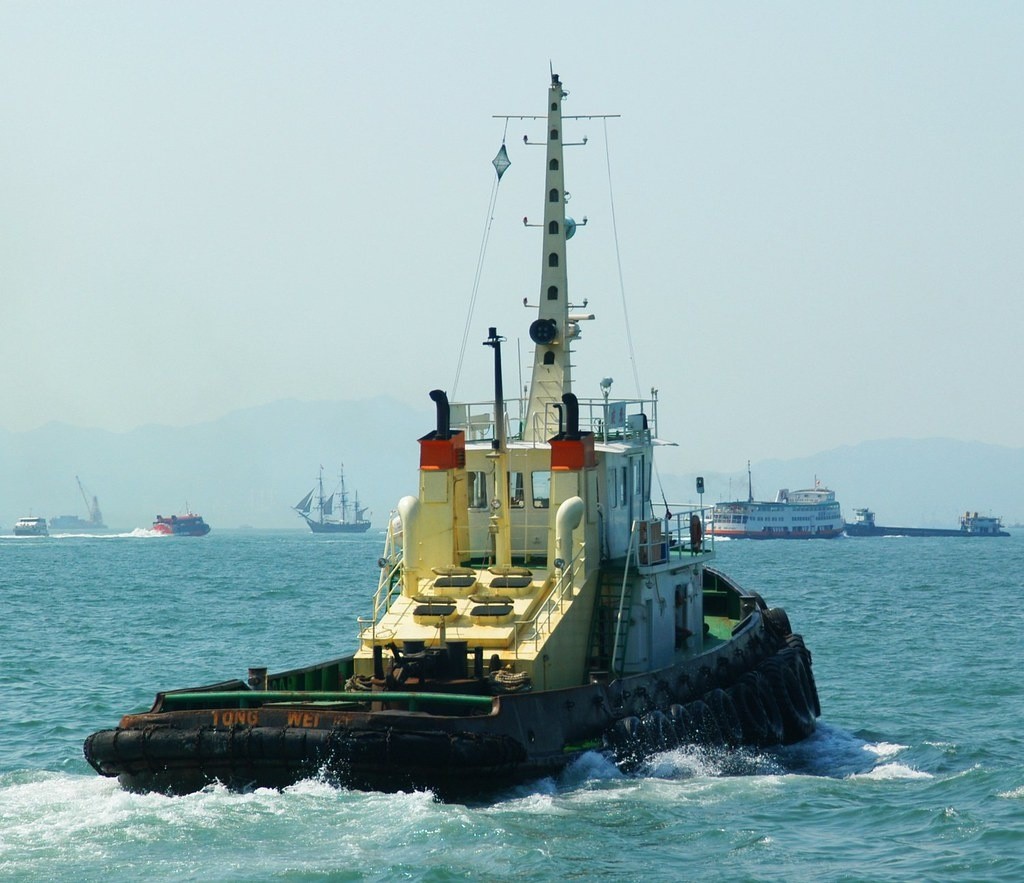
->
<box><xmin>704</xmin><ymin>460</ymin><xmax>847</xmax><ymax>540</ymax></box>
<box><xmin>843</xmin><ymin>506</ymin><xmax>888</xmax><ymax>537</ymax></box>
<box><xmin>13</xmin><ymin>513</ymin><xmax>49</xmax><ymax>536</ymax></box>
<box><xmin>950</xmin><ymin>510</ymin><xmax>1011</xmax><ymax>537</ymax></box>
<box><xmin>82</xmin><ymin>60</ymin><xmax>821</xmax><ymax>812</ymax></box>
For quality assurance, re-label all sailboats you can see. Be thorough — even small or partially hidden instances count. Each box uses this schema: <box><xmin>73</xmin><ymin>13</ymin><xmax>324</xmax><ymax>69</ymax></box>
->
<box><xmin>290</xmin><ymin>459</ymin><xmax>372</xmax><ymax>534</ymax></box>
<box><xmin>147</xmin><ymin>497</ymin><xmax>211</xmax><ymax>536</ymax></box>
